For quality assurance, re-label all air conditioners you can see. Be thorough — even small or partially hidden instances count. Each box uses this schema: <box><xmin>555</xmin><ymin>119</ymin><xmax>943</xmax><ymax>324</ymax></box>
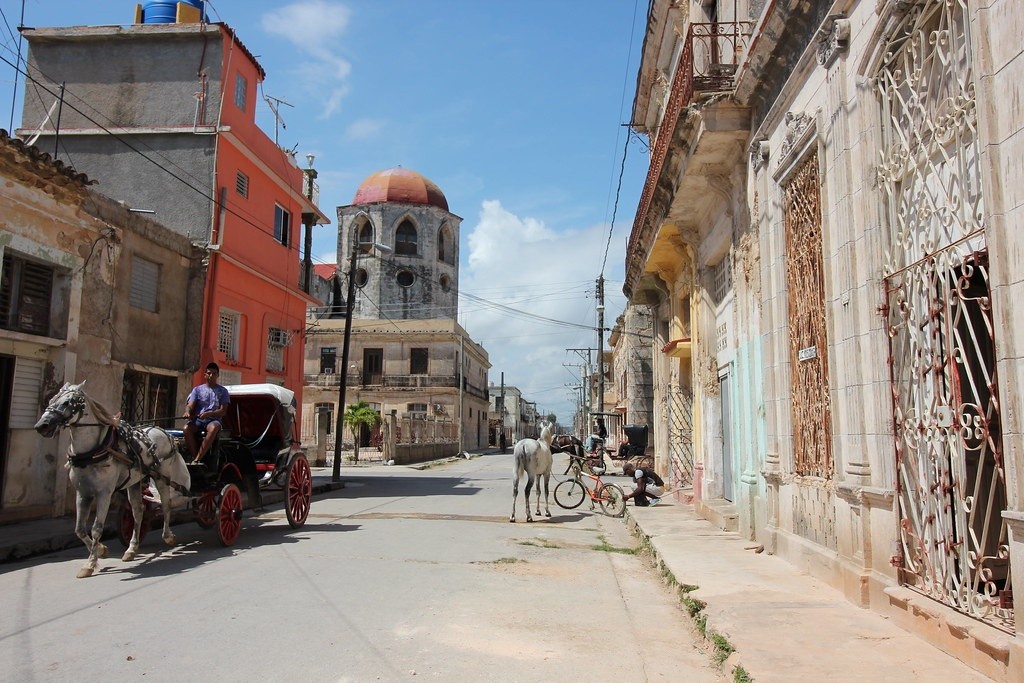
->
<box><xmin>432</xmin><ymin>404</ymin><xmax>442</xmax><ymax>412</ymax></box>
<box><xmin>274</xmin><ymin>331</ymin><xmax>288</xmax><ymax>346</ymax></box>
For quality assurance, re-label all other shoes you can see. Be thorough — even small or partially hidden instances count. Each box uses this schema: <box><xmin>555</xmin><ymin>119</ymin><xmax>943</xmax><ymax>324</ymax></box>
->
<box><xmin>191</xmin><ymin>461</ymin><xmax>199</xmax><ymax>465</ymax></box>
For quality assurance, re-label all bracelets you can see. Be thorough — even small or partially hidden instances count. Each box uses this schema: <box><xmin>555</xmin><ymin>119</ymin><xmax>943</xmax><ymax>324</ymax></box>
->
<box><xmin>628</xmin><ymin>495</ymin><xmax>629</xmax><ymax>498</ymax></box>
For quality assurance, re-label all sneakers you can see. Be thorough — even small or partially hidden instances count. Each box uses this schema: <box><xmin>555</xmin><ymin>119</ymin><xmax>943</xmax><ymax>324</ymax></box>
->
<box><xmin>649</xmin><ymin>497</ymin><xmax>662</xmax><ymax>507</ymax></box>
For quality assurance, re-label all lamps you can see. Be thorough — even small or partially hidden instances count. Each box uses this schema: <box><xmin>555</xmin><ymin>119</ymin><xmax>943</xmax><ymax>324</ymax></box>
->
<box><xmin>306</xmin><ymin>153</ymin><xmax>315</xmax><ymax>169</ymax></box>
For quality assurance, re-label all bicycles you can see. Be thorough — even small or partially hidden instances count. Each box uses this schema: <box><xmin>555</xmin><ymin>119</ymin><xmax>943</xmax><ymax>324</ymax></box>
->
<box><xmin>554</xmin><ymin>457</ymin><xmax>626</xmax><ymax>518</ymax></box>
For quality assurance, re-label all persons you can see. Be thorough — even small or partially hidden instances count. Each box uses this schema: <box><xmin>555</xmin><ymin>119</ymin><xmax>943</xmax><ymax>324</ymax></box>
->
<box><xmin>586</xmin><ymin>419</ymin><xmax>607</xmax><ymax>451</ymax></box>
<box><xmin>183</xmin><ymin>362</ymin><xmax>230</xmax><ymax>463</ymax></box>
<box><xmin>500</xmin><ymin>433</ymin><xmax>505</xmax><ymax>449</ymax></box>
<box><xmin>622</xmin><ymin>463</ymin><xmax>665</xmax><ymax>506</ymax></box>
<box><xmin>616</xmin><ymin>438</ymin><xmax>630</xmax><ymax>459</ymax></box>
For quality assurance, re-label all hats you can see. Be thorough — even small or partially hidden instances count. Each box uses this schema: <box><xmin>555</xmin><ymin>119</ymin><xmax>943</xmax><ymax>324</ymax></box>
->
<box><xmin>624</xmin><ymin>463</ymin><xmax>633</xmax><ymax>474</ymax></box>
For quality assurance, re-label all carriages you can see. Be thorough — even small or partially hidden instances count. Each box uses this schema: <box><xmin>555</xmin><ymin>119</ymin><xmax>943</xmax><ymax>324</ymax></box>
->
<box><xmin>34</xmin><ymin>379</ymin><xmax>313</xmax><ymax>579</ymax></box>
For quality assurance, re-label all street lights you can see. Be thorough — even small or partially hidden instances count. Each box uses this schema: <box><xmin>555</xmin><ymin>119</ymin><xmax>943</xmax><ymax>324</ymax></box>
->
<box><xmin>458</xmin><ymin>334</ymin><xmax>465</xmax><ymax>454</ymax></box>
<box><xmin>332</xmin><ymin>223</ymin><xmax>392</xmax><ymax>481</ymax></box>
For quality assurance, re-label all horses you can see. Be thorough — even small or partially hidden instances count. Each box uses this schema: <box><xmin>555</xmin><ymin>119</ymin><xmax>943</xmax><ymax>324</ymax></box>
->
<box><xmin>529</xmin><ymin>434</ymin><xmax>584</xmax><ymax>475</ymax></box>
<box><xmin>509</xmin><ymin>422</ymin><xmax>554</xmax><ymax>522</ymax></box>
<box><xmin>34</xmin><ymin>380</ymin><xmax>176</xmax><ymax>578</ymax></box>
